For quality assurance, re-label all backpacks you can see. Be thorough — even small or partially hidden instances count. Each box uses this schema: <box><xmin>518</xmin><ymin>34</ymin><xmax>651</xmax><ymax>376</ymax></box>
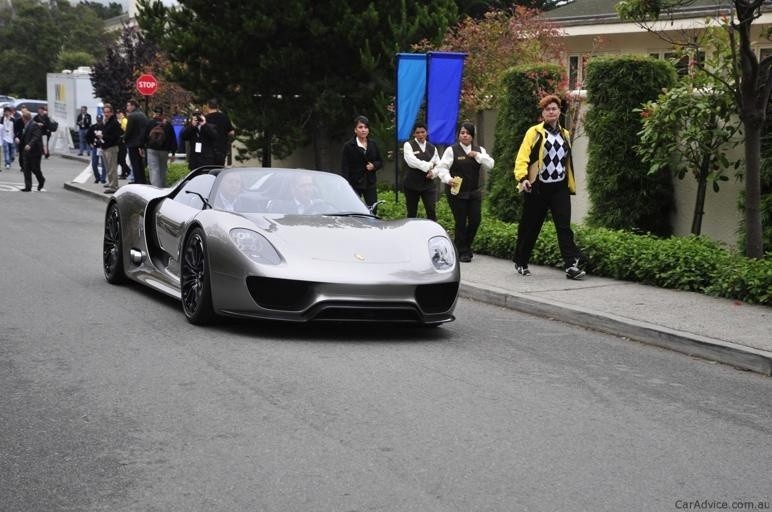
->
<box><xmin>148</xmin><ymin>125</ymin><xmax>166</xmax><ymax>150</ymax></box>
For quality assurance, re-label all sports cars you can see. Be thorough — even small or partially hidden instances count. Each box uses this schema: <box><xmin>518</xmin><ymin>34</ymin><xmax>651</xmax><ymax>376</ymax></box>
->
<box><xmin>103</xmin><ymin>162</ymin><xmax>460</xmax><ymax>332</ymax></box>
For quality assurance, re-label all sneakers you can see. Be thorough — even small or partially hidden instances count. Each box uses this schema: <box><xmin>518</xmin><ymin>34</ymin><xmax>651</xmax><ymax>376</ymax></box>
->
<box><xmin>38</xmin><ymin>178</ymin><xmax>45</xmax><ymax>191</ymax></box>
<box><xmin>104</xmin><ymin>189</ymin><xmax>118</xmax><ymax>194</ymax></box>
<box><xmin>565</xmin><ymin>267</ymin><xmax>586</xmax><ymax>278</ymax></box>
<box><xmin>515</xmin><ymin>264</ymin><xmax>533</xmax><ymax>276</ymax></box>
<box><xmin>459</xmin><ymin>251</ymin><xmax>473</xmax><ymax>262</ymax></box>
<box><xmin>95</xmin><ymin>179</ymin><xmax>100</xmax><ymax>184</ymax></box>
<box><xmin>21</xmin><ymin>188</ymin><xmax>31</xmax><ymax>192</ymax></box>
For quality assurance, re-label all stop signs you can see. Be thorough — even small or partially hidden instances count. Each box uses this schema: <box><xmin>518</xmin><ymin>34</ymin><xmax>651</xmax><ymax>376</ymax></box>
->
<box><xmin>136</xmin><ymin>74</ymin><xmax>157</xmax><ymax>95</ymax></box>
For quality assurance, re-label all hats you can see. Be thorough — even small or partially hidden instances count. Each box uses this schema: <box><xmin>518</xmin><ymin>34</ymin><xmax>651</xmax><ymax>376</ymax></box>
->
<box><xmin>152</xmin><ymin>106</ymin><xmax>163</xmax><ymax>114</ymax></box>
<box><xmin>192</xmin><ymin>108</ymin><xmax>203</xmax><ymax>115</ymax></box>
<box><xmin>96</xmin><ymin>114</ymin><xmax>103</xmax><ymax>121</ymax></box>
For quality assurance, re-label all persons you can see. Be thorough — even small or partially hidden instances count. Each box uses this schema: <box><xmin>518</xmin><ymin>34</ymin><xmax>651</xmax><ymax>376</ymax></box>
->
<box><xmin>76</xmin><ymin>97</ymin><xmax>235</xmax><ymax>193</ymax></box>
<box><xmin>513</xmin><ymin>96</ymin><xmax>587</xmax><ymax>278</ymax></box>
<box><xmin>402</xmin><ymin>124</ymin><xmax>440</xmax><ymax>221</ymax></box>
<box><xmin>207</xmin><ymin>172</ymin><xmax>259</xmax><ymax>213</ymax></box>
<box><xmin>342</xmin><ymin>115</ymin><xmax>384</xmax><ymax>216</ymax></box>
<box><xmin>441</xmin><ymin>121</ymin><xmax>496</xmax><ymax>260</ymax></box>
<box><xmin>269</xmin><ymin>177</ymin><xmax>323</xmax><ymax>214</ymax></box>
<box><xmin>1</xmin><ymin>104</ymin><xmax>53</xmax><ymax>192</ymax></box>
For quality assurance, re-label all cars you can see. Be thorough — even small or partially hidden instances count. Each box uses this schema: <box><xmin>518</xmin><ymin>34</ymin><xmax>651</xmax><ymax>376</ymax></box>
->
<box><xmin>1</xmin><ymin>95</ymin><xmax>17</xmax><ymax>106</ymax></box>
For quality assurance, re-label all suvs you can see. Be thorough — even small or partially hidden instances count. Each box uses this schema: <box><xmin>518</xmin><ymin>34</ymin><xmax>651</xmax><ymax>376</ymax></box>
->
<box><xmin>1</xmin><ymin>100</ymin><xmax>49</xmax><ymax>120</ymax></box>
